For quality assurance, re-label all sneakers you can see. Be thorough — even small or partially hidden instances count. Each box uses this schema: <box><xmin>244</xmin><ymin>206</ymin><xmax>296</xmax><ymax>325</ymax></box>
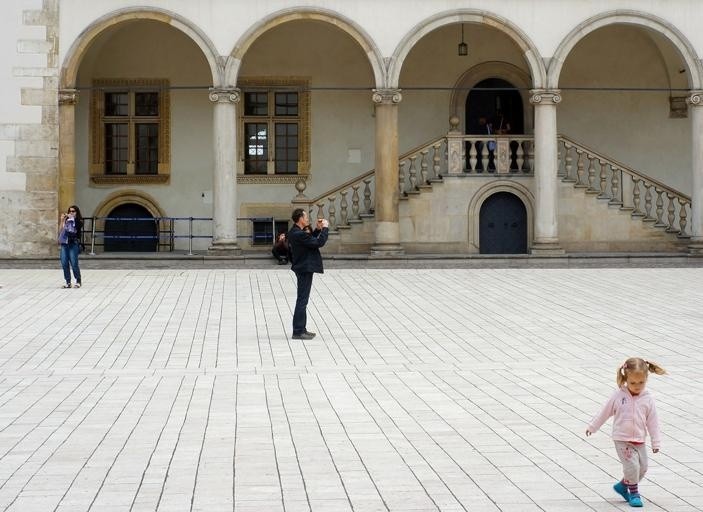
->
<box><xmin>292</xmin><ymin>333</ymin><xmax>313</xmax><ymax>339</ymax></box>
<box><xmin>62</xmin><ymin>283</ymin><xmax>72</xmax><ymax>288</ymax></box>
<box><xmin>628</xmin><ymin>492</ymin><xmax>642</xmax><ymax>507</ymax></box>
<box><xmin>304</xmin><ymin>328</ymin><xmax>315</xmax><ymax>336</ymax></box>
<box><xmin>73</xmin><ymin>283</ymin><xmax>80</xmax><ymax>288</ymax></box>
<box><xmin>613</xmin><ymin>482</ymin><xmax>628</xmax><ymax>501</ymax></box>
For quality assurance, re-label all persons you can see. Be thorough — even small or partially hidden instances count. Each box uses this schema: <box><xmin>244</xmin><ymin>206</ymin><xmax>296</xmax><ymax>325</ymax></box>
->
<box><xmin>585</xmin><ymin>358</ymin><xmax>667</xmax><ymax>508</ymax></box>
<box><xmin>285</xmin><ymin>208</ymin><xmax>330</xmax><ymax>342</ymax></box>
<box><xmin>271</xmin><ymin>232</ymin><xmax>291</xmax><ymax>266</ymax></box>
<box><xmin>58</xmin><ymin>205</ymin><xmax>85</xmax><ymax>289</ymax></box>
<box><xmin>466</xmin><ymin>108</ymin><xmax>514</xmax><ymax>173</ymax></box>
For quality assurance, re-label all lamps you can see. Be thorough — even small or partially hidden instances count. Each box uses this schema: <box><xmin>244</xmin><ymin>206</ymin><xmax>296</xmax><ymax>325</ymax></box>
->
<box><xmin>458</xmin><ymin>23</ymin><xmax>468</xmax><ymax>56</ymax></box>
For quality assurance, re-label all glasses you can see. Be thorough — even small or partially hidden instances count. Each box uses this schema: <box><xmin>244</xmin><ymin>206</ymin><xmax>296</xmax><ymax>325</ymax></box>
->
<box><xmin>69</xmin><ymin>211</ymin><xmax>75</xmax><ymax>212</ymax></box>
<box><xmin>303</xmin><ymin>228</ymin><xmax>308</xmax><ymax>231</ymax></box>
